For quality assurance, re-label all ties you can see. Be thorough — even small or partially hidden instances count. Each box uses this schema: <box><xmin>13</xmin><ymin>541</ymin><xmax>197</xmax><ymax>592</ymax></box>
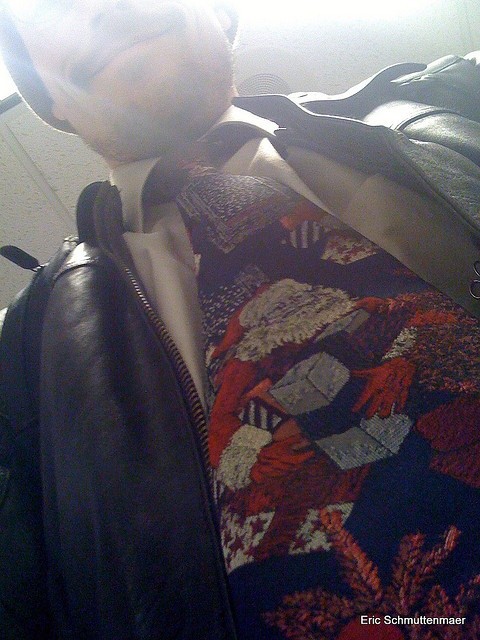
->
<box><xmin>143</xmin><ymin>126</ymin><xmax>480</xmax><ymax>640</ymax></box>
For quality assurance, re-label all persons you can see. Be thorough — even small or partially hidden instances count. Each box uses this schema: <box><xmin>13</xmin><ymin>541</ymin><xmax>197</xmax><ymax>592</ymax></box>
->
<box><xmin>0</xmin><ymin>0</ymin><xmax>480</xmax><ymax>640</ymax></box>
